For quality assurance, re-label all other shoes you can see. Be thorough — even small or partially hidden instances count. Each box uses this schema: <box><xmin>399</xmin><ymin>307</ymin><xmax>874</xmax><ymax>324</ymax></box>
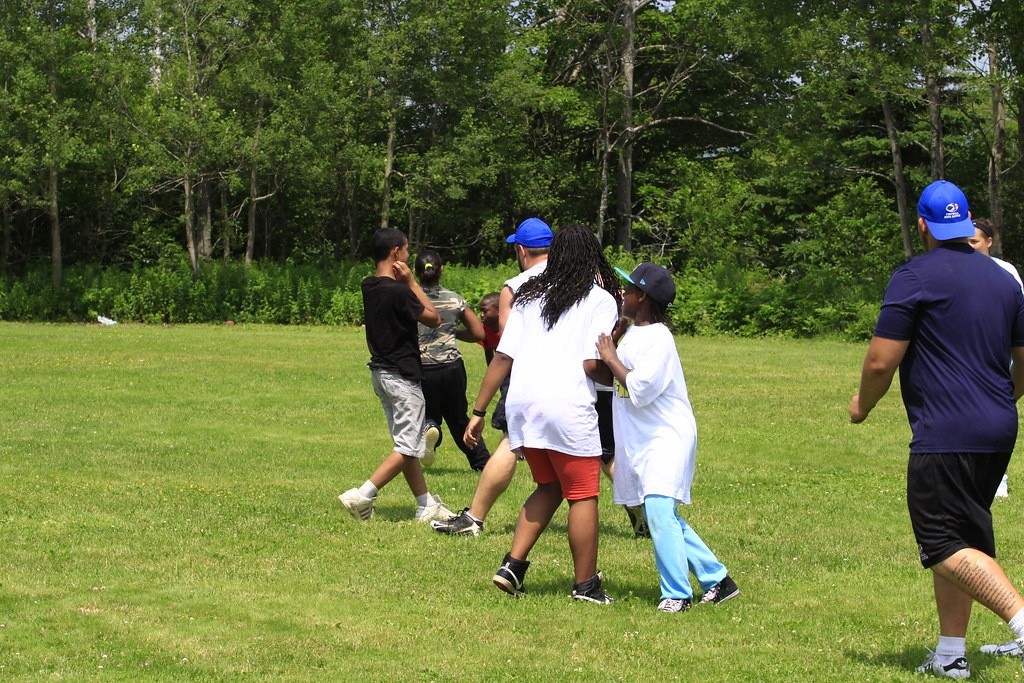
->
<box><xmin>993</xmin><ymin>475</ymin><xmax>1007</xmax><ymax>498</ymax></box>
<box><xmin>420</xmin><ymin>421</ymin><xmax>440</xmax><ymax>467</ymax></box>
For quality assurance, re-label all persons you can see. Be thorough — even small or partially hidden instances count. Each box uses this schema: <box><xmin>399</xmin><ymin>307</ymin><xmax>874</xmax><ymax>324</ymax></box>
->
<box><xmin>851</xmin><ymin>179</ymin><xmax>1024</xmax><ymax>679</ymax></box>
<box><xmin>339</xmin><ymin>228</ymin><xmax>454</xmax><ymax>523</ymax></box>
<box><xmin>478</xmin><ymin>291</ymin><xmax>500</xmax><ymax>366</ymax></box>
<box><xmin>595</xmin><ymin>263</ymin><xmax>740</xmax><ymax>610</ymax></box>
<box><xmin>414</xmin><ymin>249</ymin><xmax>490</xmax><ymax>471</ymax></box>
<box><xmin>462</xmin><ymin>223</ymin><xmax>617</xmax><ymax>605</ymax></box>
<box><xmin>430</xmin><ymin>217</ymin><xmax>650</xmax><ymax>538</ymax></box>
<box><xmin>969</xmin><ymin>219</ymin><xmax>1024</xmax><ymax>498</ymax></box>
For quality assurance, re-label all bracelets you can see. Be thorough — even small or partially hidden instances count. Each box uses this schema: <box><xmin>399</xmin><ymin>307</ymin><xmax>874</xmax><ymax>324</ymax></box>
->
<box><xmin>473</xmin><ymin>409</ymin><xmax>486</xmax><ymax>416</ymax></box>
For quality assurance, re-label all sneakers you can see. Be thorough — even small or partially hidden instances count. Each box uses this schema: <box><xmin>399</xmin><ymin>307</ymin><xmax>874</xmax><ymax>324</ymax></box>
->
<box><xmin>655</xmin><ymin>599</ymin><xmax>692</xmax><ymax>616</ymax></box>
<box><xmin>431</xmin><ymin>507</ymin><xmax>482</xmax><ymax>537</ymax></box>
<box><xmin>623</xmin><ymin>502</ymin><xmax>652</xmax><ymax>538</ymax></box>
<box><xmin>413</xmin><ymin>501</ymin><xmax>455</xmax><ymax>522</ymax></box>
<box><xmin>696</xmin><ymin>573</ymin><xmax>739</xmax><ymax>606</ymax></box>
<box><xmin>915</xmin><ymin>644</ymin><xmax>970</xmax><ymax>680</ymax></box>
<box><xmin>494</xmin><ymin>552</ymin><xmax>531</xmax><ymax>597</ymax></box>
<box><xmin>339</xmin><ymin>486</ymin><xmax>377</xmax><ymax>522</ymax></box>
<box><xmin>570</xmin><ymin>569</ymin><xmax>615</xmax><ymax>606</ymax></box>
<box><xmin>980</xmin><ymin>642</ymin><xmax>1024</xmax><ymax>660</ymax></box>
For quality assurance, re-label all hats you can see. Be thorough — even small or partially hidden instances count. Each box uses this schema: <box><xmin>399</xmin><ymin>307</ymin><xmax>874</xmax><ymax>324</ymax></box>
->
<box><xmin>613</xmin><ymin>261</ymin><xmax>677</xmax><ymax>307</ymax></box>
<box><xmin>506</xmin><ymin>218</ymin><xmax>554</xmax><ymax>247</ymax></box>
<box><xmin>917</xmin><ymin>180</ymin><xmax>976</xmax><ymax>240</ymax></box>
